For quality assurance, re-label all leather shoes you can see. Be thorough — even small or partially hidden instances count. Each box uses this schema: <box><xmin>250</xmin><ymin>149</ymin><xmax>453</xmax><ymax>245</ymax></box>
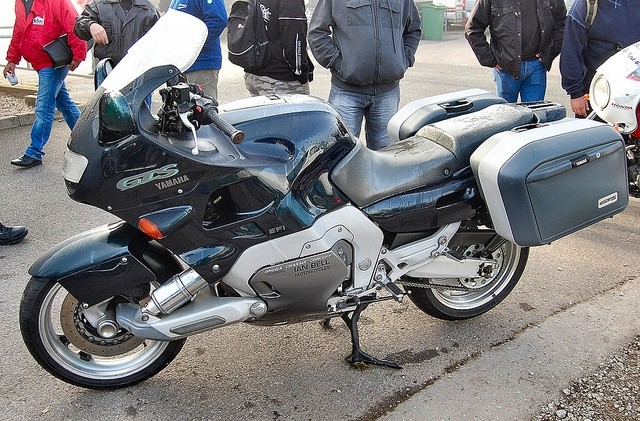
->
<box><xmin>11</xmin><ymin>155</ymin><xmax>42</xmax><ymax>167</ymax></box>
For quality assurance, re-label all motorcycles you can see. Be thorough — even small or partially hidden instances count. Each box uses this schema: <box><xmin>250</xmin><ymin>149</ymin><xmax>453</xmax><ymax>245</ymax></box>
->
<box><xmin>20</xmin><ymin>9</ymin><xmax>630</xmax><ymax>391</ymax></box>
<box><xmin>585</xmin><ymin>42</ymin><xmax>640</xmax><ymax>199</ymax></box>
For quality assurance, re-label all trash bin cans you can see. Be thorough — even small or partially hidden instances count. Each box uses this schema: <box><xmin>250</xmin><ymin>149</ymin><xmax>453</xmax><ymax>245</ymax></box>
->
<box><xmin>422</xmin><ymin>3</ymin><xmax>447</xmax><ymax>40</ymax></box>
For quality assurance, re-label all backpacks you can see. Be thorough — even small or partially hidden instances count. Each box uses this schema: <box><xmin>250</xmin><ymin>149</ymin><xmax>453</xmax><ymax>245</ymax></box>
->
<box><xmin>227</xmin><ymin>0</ymin><xmax>275</xmax><ymax>69</ymax></box>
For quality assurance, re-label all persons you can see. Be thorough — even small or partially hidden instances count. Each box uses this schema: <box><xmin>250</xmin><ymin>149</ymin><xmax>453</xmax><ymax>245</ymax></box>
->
<box><xmin>560</xmin><ymin>0</ymin><xmax>636</xmax><ymax>120</ymax></box>
<box><xmin>169</xmin><ymin>0</ymin><xmax>228</xmax><ymax>101</ymax></box>
<box><xmin>2</xmin><ymin>1</ymin><xmax>88</xmax><ymax>169</ymax></box>
<box><xmin>243</xmin><ymin>1</ymin><xmax>314</xmax><ymax>100</ymax></box>
<box><xmin>464</xmin><ymin>0</ymin><xmax>567</xmax><ymax>102</ymax></box>
<box><xmin>307</xmin><ymin>1</ymin><xmax>421</xmax><ymax>149</ymax></box>
<box><xmin>74</xmin><ymin>1</ymin><xmax>160</xmax><ymax>112</ymax></box>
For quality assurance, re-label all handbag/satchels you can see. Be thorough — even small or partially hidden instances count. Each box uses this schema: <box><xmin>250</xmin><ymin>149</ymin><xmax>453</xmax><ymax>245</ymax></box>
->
<box><xmin>41</xmin><ymin>33</ymin><xmax>94</xmax><ymax>69</ymax></box>
